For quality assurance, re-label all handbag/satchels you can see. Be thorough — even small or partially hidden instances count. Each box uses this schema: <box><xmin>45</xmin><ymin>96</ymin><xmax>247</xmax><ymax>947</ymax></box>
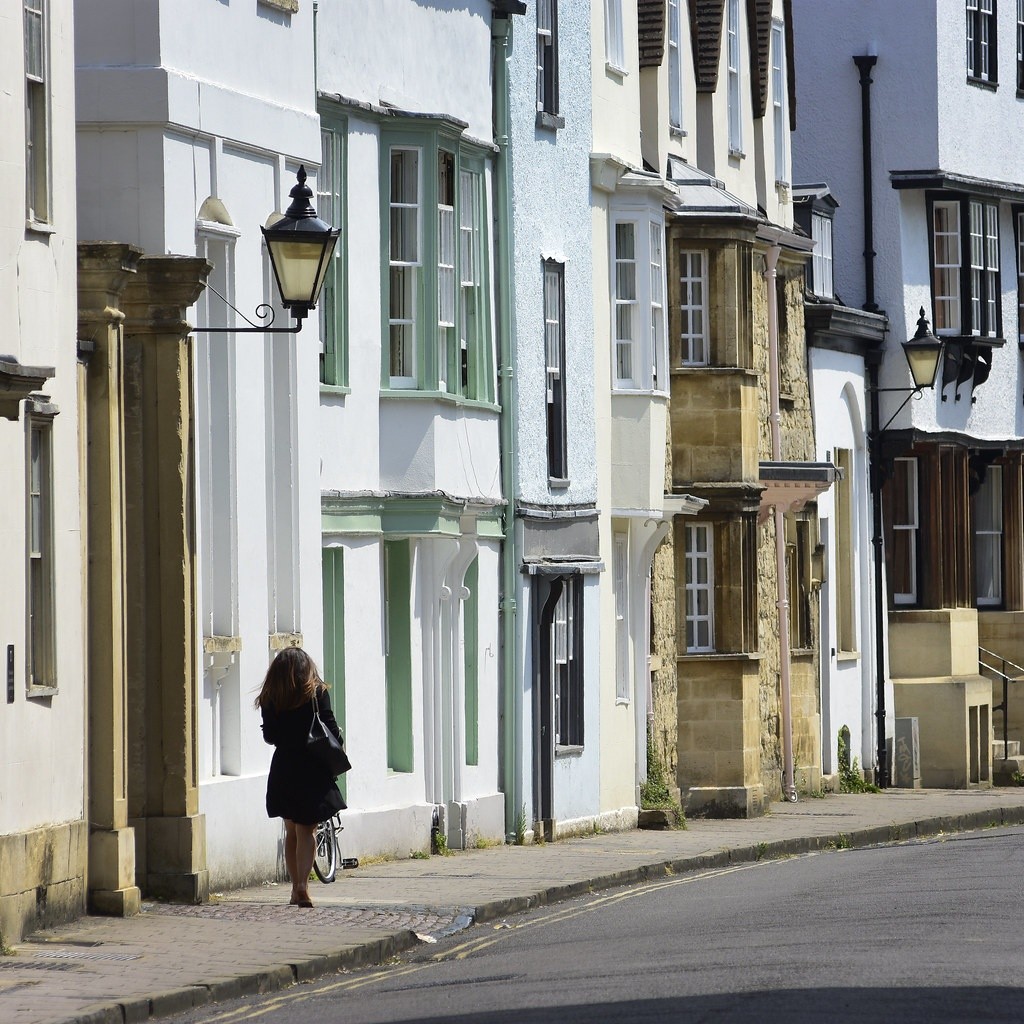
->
<box><xmin>308</xmin><ymin>685</ymin><xmax>352</xmax><ymax>775</ymax></box>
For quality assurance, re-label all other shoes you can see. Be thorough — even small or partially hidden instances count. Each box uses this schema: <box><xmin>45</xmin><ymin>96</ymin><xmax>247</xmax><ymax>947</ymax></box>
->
<box><xmin>290</xmin><ymin>901</ymin><xmax>297</xmax><ymax>904</ymax></box>
<box><xmin>298</xmin><ymin>900</ymin><xmax>312</xmax><ymax>908</ymax></box>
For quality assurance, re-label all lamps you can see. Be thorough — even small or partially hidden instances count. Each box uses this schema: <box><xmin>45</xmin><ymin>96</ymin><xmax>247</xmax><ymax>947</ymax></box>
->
<box><xmin>865</xmin><ymin>305</ymin><xmax>948</xmax><ymax>442</ymax></box>
<box><xmin>189</xmin><ymin>163</ymin><xmax>340</xmax><ymax>334</ymax></box>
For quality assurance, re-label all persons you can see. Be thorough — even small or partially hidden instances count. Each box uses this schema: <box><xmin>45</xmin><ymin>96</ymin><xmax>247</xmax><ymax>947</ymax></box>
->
<box><xmin>254</xmin><ymin>647</ymin><xmax>348</xmax><ymax>908</ymax></box>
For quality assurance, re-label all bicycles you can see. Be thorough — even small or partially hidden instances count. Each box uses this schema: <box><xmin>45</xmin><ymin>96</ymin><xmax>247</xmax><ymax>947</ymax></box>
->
<box><xmin>312</xmin><ymin>807</ymin><xmax>359</xmax><ymax>884</ymax></box>
